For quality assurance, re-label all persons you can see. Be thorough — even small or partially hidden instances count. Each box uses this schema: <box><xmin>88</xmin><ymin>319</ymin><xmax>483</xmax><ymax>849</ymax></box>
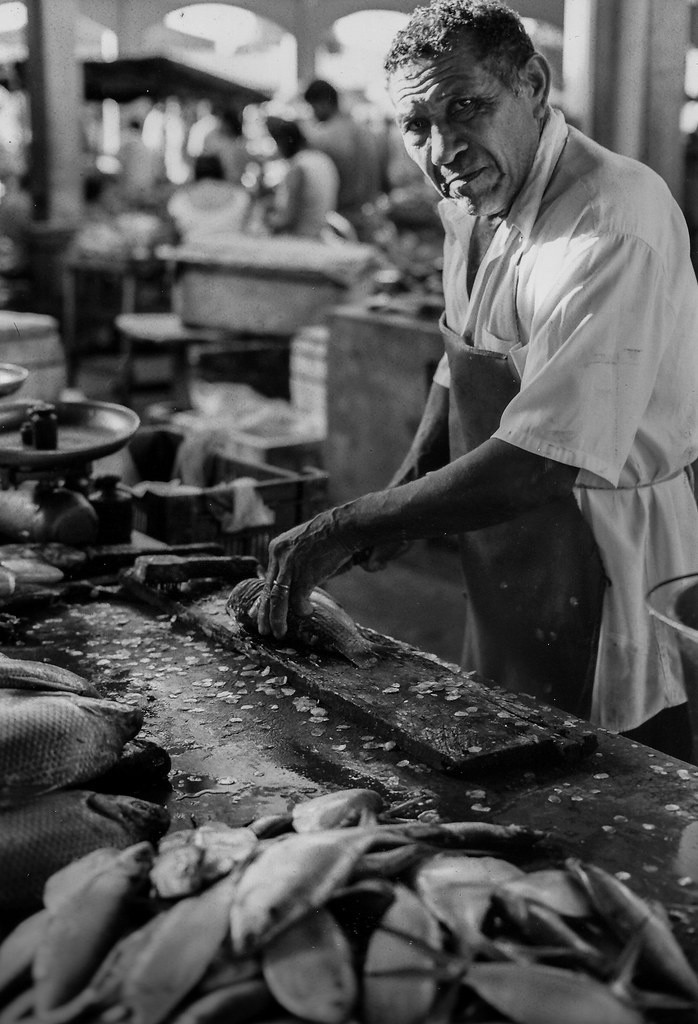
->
<box><xmin>77</xmin><ymin>79</ymin><xmax>374</xmax><ymax>343</ymax></box>
<box><xmin>255</xmin><ymin>0</ymin><xmax>698</xmax><ymax>761</ymax></box>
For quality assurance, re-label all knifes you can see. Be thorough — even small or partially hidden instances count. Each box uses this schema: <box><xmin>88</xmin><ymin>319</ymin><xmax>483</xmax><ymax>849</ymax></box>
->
<box><xmin>329</xmin><ymin>546</ymin><xmax>374</xmax><ymax>578</ymax></box>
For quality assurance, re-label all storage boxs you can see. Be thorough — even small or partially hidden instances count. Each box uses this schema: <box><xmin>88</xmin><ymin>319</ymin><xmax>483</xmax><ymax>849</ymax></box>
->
<box><xmin>93</xmin><ymin>429</ymin><xmax>300</xmax><ymax>565</ymax></box>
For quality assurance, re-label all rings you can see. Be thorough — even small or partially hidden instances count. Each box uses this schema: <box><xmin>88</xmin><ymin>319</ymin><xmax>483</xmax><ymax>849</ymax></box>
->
<box><xmin>273</xmin><ymin>580</ymin><xmax>290</xmax><ymax>590</ymax></box>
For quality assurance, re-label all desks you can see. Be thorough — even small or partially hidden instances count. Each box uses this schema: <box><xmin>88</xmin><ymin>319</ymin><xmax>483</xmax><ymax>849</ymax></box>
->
<box><xmin>59</xmin><ymin>569</ymin><xmax>698</xmax><ymax>977</ymax></box>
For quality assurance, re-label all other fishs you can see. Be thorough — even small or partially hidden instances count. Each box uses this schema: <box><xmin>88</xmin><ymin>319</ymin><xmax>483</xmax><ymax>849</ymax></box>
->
<box><xmin>0</xmin><ymin>638</ymin><xmax>190</xmax><ymax>783</ymax></box>
<box><xmin>0</xmin><ymin>785</ymin><xmax>698</xmax><ymax>1023</ymax></box>
<box><xmin>223</xmin><ymin>575</ymin><xmax>393</xmax><ymax>670</ymax></box>
